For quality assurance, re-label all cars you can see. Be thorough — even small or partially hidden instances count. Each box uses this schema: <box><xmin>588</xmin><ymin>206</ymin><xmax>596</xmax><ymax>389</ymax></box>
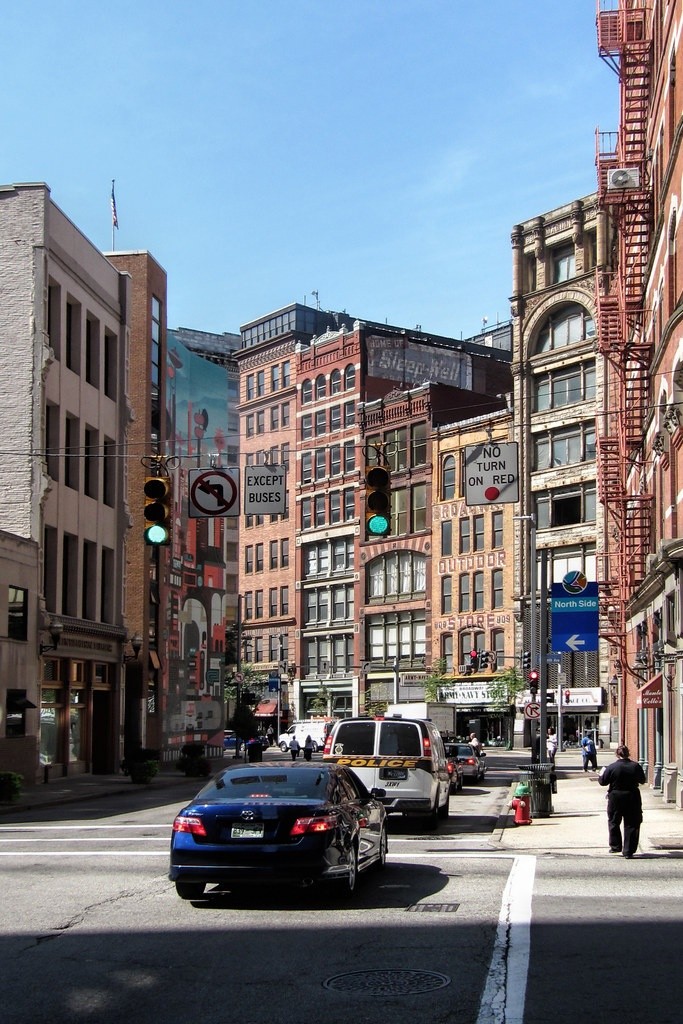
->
<box><xmin>168</xmin><ymin>761</ymin><xmax>390</xmax><ymax>900</ymax></box>
<box><xmin>444</xmin><ymin>742</ymin><xmax>489</xmax><ymax>786</ymax></box>
<box><xmin>223</xmin><ymin>732</ymin><xmax>270</xmax><ymax>751</ymax></box>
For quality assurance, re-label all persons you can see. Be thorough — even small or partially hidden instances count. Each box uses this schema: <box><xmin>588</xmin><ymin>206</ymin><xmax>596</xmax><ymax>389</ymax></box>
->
<box><xmin>289</xmin><ymin>736</ymin><xmax>299</xmax><ymax>762</ymax></box>
<box><xmin>580</xmin><ymin>731</ymin><xmax>599</xmax><ymax>773</ymax></box>
<box><xmin>303</xmin><ymin>735</ymin><xmax>315</xmax><ymax>762</ymax></box>
<box><xmin>545</xmin><ymin>727</ymin><xmax>557</xmax><ymax>771</ymax></box>
<box><xmin>535</xmin><ymin>726</ymin><xmax>540</xmax><ymax>757</ymax></box>
<box><xmin>468</xmin><ymin>732</ymin><xmax>482</xmax><ymax>756</ymax></box>
<box><xmin>249</xmin><ymin>737</ymin><xmax>255</xmax><ymax>745</ymax></box>
<box><xmin>265</xmin><ymin>725</ymin><xmax>274</xmax><ymax>746</ymax></box>
<box><xmin>598</xmin><ymin>745</ymin><xmax>646</xmax><ymax>859</ymax></box>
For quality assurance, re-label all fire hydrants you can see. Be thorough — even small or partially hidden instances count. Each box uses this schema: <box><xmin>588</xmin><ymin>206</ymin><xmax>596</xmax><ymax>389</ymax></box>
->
<box><xmin>509</xmin><ymin>782</ymin><xmax>533</xmax><ymax>825</ymax></box>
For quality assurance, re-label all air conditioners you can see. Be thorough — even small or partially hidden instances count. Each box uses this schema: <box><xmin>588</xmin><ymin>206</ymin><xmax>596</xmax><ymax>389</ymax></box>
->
<box><xmin>607</xmin><ymin>167</ymin><xmax>640</xmax><ymax>192</ymax></box>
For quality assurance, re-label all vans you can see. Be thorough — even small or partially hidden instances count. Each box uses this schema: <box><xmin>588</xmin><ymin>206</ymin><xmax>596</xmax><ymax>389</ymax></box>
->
<box><xmin>278</xmin><ymin>719</ymin><xmax>333</xmax><ymax>753</ymax></box>
<box><xmin>322</xmin><ymin>712</ymin><xmax>459</xmax><ymax>831</ymax></box>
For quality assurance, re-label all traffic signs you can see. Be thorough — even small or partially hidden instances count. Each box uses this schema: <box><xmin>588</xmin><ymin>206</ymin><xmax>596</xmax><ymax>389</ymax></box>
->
<box><xmin>550</xmin><ymin>582</ymin><xmax>600</xmax><ymax>653</ymax></box>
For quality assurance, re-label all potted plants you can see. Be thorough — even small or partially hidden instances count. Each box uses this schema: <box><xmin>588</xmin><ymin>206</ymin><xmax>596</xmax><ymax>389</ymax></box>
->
<box><xmin>174</xmin><ymin>744</ymin><xmax>207</xmax><ymax>777</ymax></box>
<box><xmin>120</xmin><ymin>748</ymin><xmax>162</xmax><ymax>784</ymax></box>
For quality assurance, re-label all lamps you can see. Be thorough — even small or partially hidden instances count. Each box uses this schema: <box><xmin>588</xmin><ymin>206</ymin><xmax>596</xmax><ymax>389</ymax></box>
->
<box><xmin>124</xmin><ymin>632</ymin><xmax>143</xmax><ymax>663</ymax></box>
<box><xmin>40</xmin><ymin>619</ymin><xmax>63</xmax><ymax>655</ymax></box>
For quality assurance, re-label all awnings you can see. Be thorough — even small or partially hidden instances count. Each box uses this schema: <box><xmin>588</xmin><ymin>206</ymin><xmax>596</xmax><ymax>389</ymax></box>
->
<box><xmin>253</xmin><ymin>698</ymin><xmax>278</xmax><ymax>717</ymax></box>
<box><xmin>636</xmin><ymin>670</ymin><xmax>662</xmax><ymax>708</ymax></box>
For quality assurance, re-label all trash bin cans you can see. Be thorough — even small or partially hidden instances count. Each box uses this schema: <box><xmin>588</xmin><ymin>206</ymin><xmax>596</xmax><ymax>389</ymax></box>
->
<box><xmin>517</xmin><ymin>763</ymin><xmax>555</xmax><ymax>817</ymax></box>
<box><xmin>247</xmin><ymin>742</ymin><xmax>263</xmax><ymax>762</ymax></box>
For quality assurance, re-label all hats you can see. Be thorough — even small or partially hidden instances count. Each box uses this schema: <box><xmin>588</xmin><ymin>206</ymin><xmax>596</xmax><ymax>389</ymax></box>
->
<box><xmin>586</xmin><ymin>731</ymin><xmax>592</xmax><ymax>736</ymax></box>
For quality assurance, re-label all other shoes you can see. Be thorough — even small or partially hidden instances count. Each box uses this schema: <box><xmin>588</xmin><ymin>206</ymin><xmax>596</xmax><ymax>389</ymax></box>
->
<box><xmin>583</xmin><ymin>769</ymin><xmax>589</xmax><ymax>773</ymax></box>
<box><xmin>592</xmin><ymin>768</ymin><xmax>596</xmax><ymax>772</ymax></box>
<box><xmin>626</xmin><ymin>854</ymin><xmax>632</xmax><ymax>858</ymax></box>
<box><xmin>609</xmin><ymin>849</ymin><xmax>618</xmax><ymax>852</ymax></box>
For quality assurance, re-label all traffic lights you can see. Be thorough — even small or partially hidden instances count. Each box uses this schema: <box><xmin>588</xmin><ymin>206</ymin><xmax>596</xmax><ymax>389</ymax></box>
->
<box><xmin>144</xmin><ymin>475</ymin><xmax>171</xmax><ymax>545</ymax></box>
<box><xmin>470</xmin><ymin>650</ymin><xmax>478</xmax><ymax>670</ymax></box>
<box><xmin>565</xmin><ymin>690</ymin><xmax>570</xmax><ymax>703</ymax></box>
<box><xmin>529</xmin><ymin>671</ymin><xmax>539</xmax><ymax>694</ymax></box>
<box><xmin>480</xmin><ymin>650</ymin><xmax>489</xmax><ymax>669</ymax></box>
<box><xmin>365</xmin><ymin>465</ymin><xmax>391</xmax><ymax>539</ymax></box>
<box><xmin>523</xmin><ymin>650</ymin><xmax>531</xmax><ymax>669</ymax></box>
